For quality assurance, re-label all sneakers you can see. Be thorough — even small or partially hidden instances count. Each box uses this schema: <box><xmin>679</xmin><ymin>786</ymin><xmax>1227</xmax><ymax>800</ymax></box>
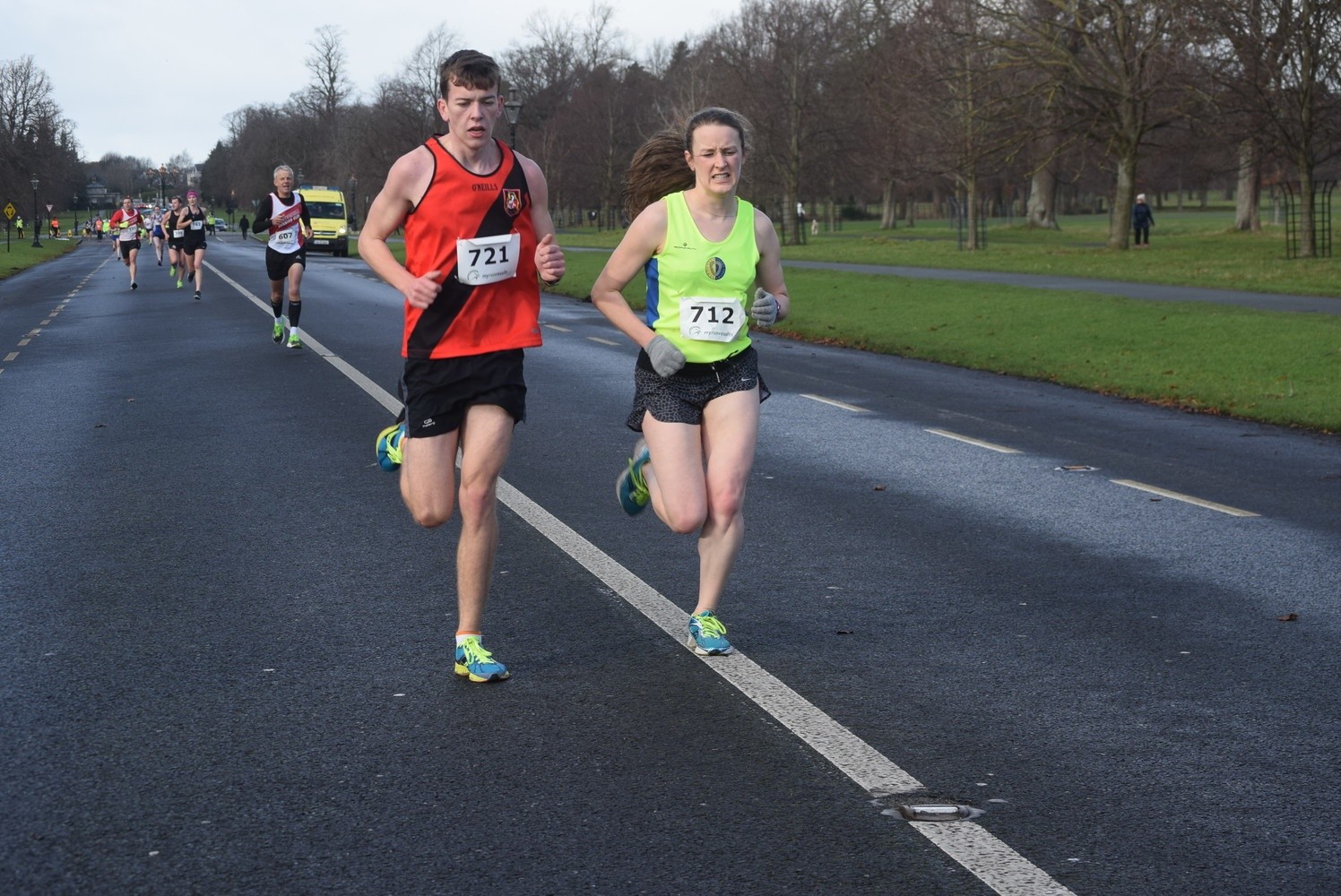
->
<box><xmin>169</xmin><ymin>266</ymin><xmax>176</xmax><ymax>278</ymax></box>
<box><xmin>286</xmin><ymin>334</ymin><xmax>301</xmax><ymax>348</ymax></box>
<box><xmin>130</xmin><ymin>281</ymin><xmax>138</xmax><ymax>290</ymax></box>
<box><xmin>454</xmin><ymin>637</ymin><xmax>511</xmax><ymax>682</ymax></box>
<box><xmin>616</xmin><ymin>437</ymin><xmax>650</xmax><ymax>516</ymax></box>
<box><xmin>687</xmin><ymin>610</ymin><xmax>735</xmax><ymax>656</ymax></box>
<box><xmin>177</xmin><ymin>280</ymin><xmax>183</xmax><ymax>289</ymax></box>
<box><xmin>193</xmin><ymin>291</ymin><xmax>201</xmax><ymax>299</ymax></box>
<box><xmin>272</xmin><ymin>315</ymin><xmax>286</xmax><ymax>345</ymax></box>
<box><xmin>187</xmin><ymin>270</ymin><xmax>195</xmax><ymax>283</ymax></box>
<box><xmin>375</xmin><ymin>419</ymin><xmax>407</xmax><ymax>472</ymax></box>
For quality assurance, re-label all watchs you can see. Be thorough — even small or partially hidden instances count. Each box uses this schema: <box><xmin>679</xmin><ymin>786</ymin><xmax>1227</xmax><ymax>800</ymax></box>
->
<box><xmin>774</xmin><ymin>299</ymin><xmax>781</xmax><ymax>324</ymax></box>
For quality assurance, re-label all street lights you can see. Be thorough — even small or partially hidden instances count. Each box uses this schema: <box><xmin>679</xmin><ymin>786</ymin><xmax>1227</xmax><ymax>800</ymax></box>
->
<box><xmin>159</xmin><ymin>162</ymin><xmax>167</xmax><ymax>209</ymax></box>
<box><xmin>146</xmin><ymin>168</ymin><xmax>155</xmax><ymax>202</ymax></box>
<box><xmin>30</xmin><ymin>173</ymin><xmax>42</xmax><ymax>247</ymax></box>
<box><xmin>347</xmin><ymin>173</ymin><xmax>358</xmax><ymax>232</ymax></box>
<box><xmin>72</xmin><ymin>193</ymin><xmax>79</xmax><ymax>236</ymax></box>
<box><xmin>501</xmin><ymin>87</ymin><xmax>522</xmax><ymax>151</ymax></box>
<box><xmin>230</xmin><ymin>189</ymin><xmax>236</xmax><ymax>232</ymax></box>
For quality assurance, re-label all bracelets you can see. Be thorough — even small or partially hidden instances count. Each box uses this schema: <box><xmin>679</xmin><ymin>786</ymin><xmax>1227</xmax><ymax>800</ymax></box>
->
<box><xmin>538</xmin><ymin>272</ymin><xmax>560</xmax><ymax>286</ymax></box>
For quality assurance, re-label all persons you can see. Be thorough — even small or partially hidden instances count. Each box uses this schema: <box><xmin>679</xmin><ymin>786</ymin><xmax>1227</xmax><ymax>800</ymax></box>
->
<box><xmin>109</xmin><ymin>198</ymin><xmax>144</xmax><ymax>290</ymax></box>
<box><xmin>239</xmin><ymin>215</ymin><xmax>249</xmax><ymax>240</ymax></box>
<box><xmin>33</xmin><ymin>215</ymin><xmax>43</xmax><ymax>237</ymax></box>
<box><xmin>252</xmin><ymin>165</ymin><xmax>314</xmax><ymax>348</ymax></box>
<box><xmin>145</xmin><ymin>188</ymin><xmax>216</xmax><ymax>300</ymax></box>
<box><xmin>358</xmin><ymin>49</ymin><xmax>565</xmax><ymax>683</ymax></box>
<box><xmin>1132</xmin><ymin>194</ymin><xmax>1155</xmax><ymax>249</ymax></box>
<box><xmin>51</xmin><ymin>217</ymin><xmax>60</xmax><ymax>238</ymax></box>
<box><xmin>103</xmin><ymin>211</ymin><xmax>145</xmax><ymax>261</ymax></box>
<box><xmin>590</xmin><ymin>107</ymin><xmax>790</xmax><ymax>656</ymax></box>
<box><xmin>86</xmin><ymin>214</ymin><xmax>103</xmax><ymax>243</ymax></box>
<box><xmin>15</xmin><ymin>216</ymin><xmax>24</xmax><ymax>239</ymax></box>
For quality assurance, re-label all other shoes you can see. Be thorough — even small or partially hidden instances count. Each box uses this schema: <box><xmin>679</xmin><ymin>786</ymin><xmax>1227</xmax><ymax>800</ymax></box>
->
<box><xmin>117</xmin><ymin>257</ymin><xmax>121</xmax><ymax>260</ymax></box>
<box><xmin>158</xmin><ymin>260</ymin><xmax>162</xmax><ymax>265</ymax></box>
<box><xmin>112</xmin><ymin>248</ymin><xmax>115</xmax><ymax>252</ymax></box>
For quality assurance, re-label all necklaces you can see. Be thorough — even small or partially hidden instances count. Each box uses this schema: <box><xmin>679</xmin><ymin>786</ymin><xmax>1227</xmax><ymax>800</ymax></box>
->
<box><xmin>694</xmin><ymin>190</ymin><xmax>738</xmax><ymax>217</ymax></box>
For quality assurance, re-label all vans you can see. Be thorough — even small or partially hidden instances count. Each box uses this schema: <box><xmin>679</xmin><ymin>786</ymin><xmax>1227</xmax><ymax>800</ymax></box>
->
<box><xmin>290</xmin><ymin>185</ymin><xmax>355</xmax><ymax>258</ymax></box>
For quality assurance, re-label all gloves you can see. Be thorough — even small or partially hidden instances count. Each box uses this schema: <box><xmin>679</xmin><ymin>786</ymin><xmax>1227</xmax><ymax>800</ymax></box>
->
<box><xmin>751</xmin><ymin>287</ymin><xmax>778</xmax><ymax>327</ymax></box>
<box><xmin>140</xmin><ymin>229</ymin><xmax>146</xmax><ymax>237</ymax></box>
<box><xmin>120</xmin><ymin>221</ymin><xmax>129</xmax><ymax>228</ymax></box>
<box><xmin>644</xmin><ymin>334</ymin><xmax>686</xmax><ymax>378</ymax></box>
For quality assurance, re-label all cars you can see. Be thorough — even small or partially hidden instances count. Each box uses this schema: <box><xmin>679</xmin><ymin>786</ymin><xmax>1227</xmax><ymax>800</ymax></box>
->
<box><xmin>214</xmin><ymin>218</ymin><xmax>228</xmax><ymax>232</ymax></box>
<box><xmin>138</xmin><ymin>203</ymin><xmax>168</xmax><ymax>218</ymax></box>
